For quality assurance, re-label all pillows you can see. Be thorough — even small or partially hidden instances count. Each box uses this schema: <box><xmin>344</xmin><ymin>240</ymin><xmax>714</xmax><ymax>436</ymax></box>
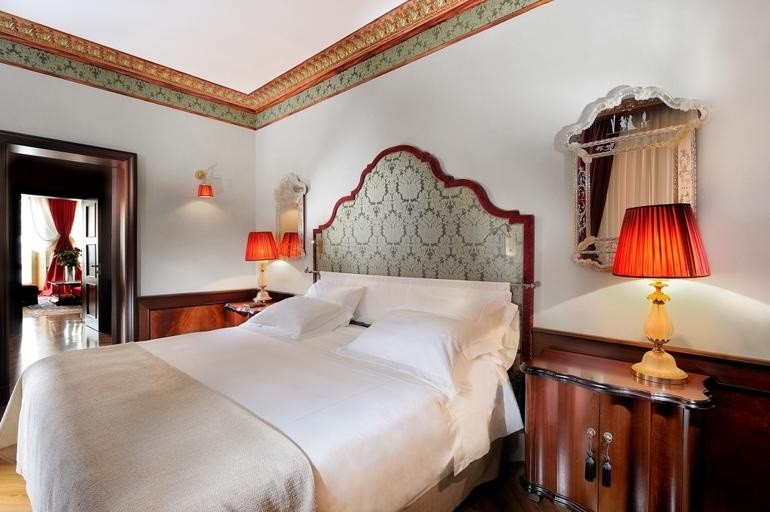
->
<box><xmin>240</xmin><ymin>295</ymin><xmax>354</xmax><ymax>344</ymax></box>
<box><xmin>332</xmin><ymin>308</ymin><xmax>475</xmax><ymax>400</ymax></box>
<box><xmin>303</xmin><ymin>279</ymin><xmax>367</xmax><ymax>328</ymax></box>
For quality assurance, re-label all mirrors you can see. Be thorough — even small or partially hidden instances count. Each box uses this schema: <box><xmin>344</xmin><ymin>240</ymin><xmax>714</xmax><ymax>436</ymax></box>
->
<box><xmin>271</xmin><ymin>174</ymin><xmax>308</xmax><ymax>256</ymax></box>
<box><xmin>556</xmin><ymin>85</ymin><xmax>709</xmax><ymax>273</ymax></box>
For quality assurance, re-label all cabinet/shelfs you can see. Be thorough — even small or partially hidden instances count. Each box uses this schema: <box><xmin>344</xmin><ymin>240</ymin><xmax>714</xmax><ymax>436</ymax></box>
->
<box><xmin>518</xmin><ymin>348</ymin><xmax>717</xmax><ymax>512</ymax></box>
<box><xmin>225</xmin><ymin>301</ymin><xmax>274</xmax><ymax>327</ymax></box>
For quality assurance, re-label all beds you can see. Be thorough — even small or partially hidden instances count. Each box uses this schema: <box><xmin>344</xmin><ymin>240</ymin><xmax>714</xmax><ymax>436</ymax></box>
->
<box><xmin>0</xmin><ymin>144</ymin><xmax>536</xmax><ymax>512</ymax></box>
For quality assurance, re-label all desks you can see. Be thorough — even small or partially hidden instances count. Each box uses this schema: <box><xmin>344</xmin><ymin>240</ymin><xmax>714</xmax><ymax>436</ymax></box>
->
<box><xmin>48</xmin><ymin>280</ymin><xmax>83</xmax><ymax>306</ymax></box>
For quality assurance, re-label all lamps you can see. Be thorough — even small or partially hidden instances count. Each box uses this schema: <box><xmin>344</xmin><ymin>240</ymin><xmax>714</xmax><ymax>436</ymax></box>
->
<box><xmin>611</xmin><ymin>201</ymin><xmax>712</xmax><ymax>384</ymax></box>
<box><xmin>195</xmin><ymin>169</ymin><xmax>213</xmax><ymax>199</ymax></box>
<box><xmin>245</xmin><ymin>231</ymin><xmax>280</xmax><ymax>303</ymax></box>
<box><xmin>280</xmin><ymin>232</ymin><xmax>304</xmax><ymax>258</ymax></box>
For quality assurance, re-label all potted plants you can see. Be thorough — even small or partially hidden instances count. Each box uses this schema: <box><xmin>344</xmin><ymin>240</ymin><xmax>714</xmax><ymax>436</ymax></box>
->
<box><xmin>58</xmin><ymin>246</ymin><xmax>82</xmax><ymax>281</ymax></box>
